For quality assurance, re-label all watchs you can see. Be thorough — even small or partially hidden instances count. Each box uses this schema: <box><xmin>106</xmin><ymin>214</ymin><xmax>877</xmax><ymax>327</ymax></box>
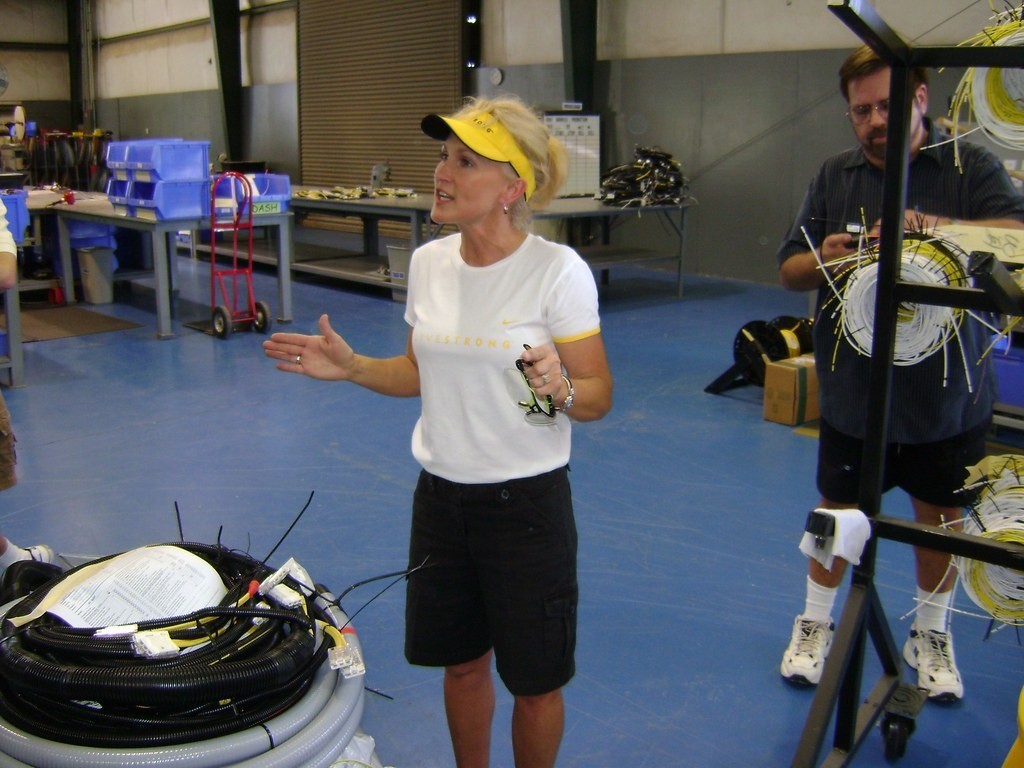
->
<box><xmin>557</xmin><ymin>375</ymin><xmax>573</xmax><ymax>413</ymax></box>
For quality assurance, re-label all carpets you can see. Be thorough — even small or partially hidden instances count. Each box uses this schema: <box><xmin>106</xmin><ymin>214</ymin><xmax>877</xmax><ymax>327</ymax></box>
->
<box><xmin>0</xmin><ymin>305</ymin><xmax>144</xmax><ymax>343</ymax></box>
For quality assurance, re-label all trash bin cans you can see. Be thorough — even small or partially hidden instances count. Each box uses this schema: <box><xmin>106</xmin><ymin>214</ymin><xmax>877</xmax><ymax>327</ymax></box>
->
<box><xmin>78</xmin><ymin>245</ymin><xmax>114</xmax><ymax>304</ymax></box>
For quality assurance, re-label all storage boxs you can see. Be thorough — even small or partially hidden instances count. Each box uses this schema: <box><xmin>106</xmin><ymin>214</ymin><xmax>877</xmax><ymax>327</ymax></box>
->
<box><xmin>763</xmin><ymin>353</ymin><xmax>821</xmax><ymax>425</ymax></box>
<box><xmin>105</xmin><ymin>139</ymin><xmax>291</xmax><ymax>243</ymax></box>
<box><xmin>989</xmin><ymin>334</ymin><xmax>1024</xmax><ymax>410</ymax></box>
<box><xmin>0</xmin><ymin>190</ymin><xmax>30</xmax><ymax>243</ymax></box>
<box><xmin>50</xmin><ymin>221</ymin><xmax>119</xmax><ymax>279</ymax></box>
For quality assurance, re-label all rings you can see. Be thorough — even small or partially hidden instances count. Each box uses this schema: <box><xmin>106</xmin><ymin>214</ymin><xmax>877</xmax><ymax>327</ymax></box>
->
<box><xmin>295</xmin><ymin>355</ymin><xmax>301</xmax><ymax>363</ymax></box>
<box><xmin>540</xmin><ymin>375</ymin><xmax>550</xmax><ymax>384</ymax></box>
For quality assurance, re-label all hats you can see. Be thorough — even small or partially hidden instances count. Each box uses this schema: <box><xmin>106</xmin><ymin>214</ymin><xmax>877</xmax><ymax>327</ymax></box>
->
<box><xmin>421</xmin><ymin>108</ymin><xmax>536</xmax><ymax>203</ymax></box>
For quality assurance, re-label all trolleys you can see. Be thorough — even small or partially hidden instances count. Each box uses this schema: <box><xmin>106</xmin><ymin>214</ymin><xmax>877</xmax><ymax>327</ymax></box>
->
<box><xmin>185</xmin><ymin>171</ymin><xmax>272</xmax><ymax>342</ymax></box>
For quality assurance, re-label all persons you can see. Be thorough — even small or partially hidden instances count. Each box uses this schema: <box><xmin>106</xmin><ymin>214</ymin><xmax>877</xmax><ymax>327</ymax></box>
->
<box><xmin>263</xmin><ymin>98</ymin><xmax>611</xmax><ymax>768</ymax></box>
<box><xmin>0</xmin><ymin>196</ymin><xmax>56</xmax><ymax>567</ymax></box>
<box><xmin>777</xmin><ymin>39</ymin><xmax>1023</xmax><ymax>701</ymax></box>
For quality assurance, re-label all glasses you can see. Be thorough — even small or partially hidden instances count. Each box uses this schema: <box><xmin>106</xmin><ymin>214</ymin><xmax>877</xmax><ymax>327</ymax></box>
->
<box><xmin>844</xmin><ymin>101</ymin><xmax>896</xmax><ymax>124</ymax></box>
<box><xmin>503</xmin><ymin>344</ymin><xmax>560</xmax><ymax>427</ymax></box>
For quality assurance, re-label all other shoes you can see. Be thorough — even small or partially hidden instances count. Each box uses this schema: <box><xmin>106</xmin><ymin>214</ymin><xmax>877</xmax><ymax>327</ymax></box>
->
<box><xmin>25</xmin><ymin>543</ymin><xmax>53</xmax><ymax>566</ymax></box>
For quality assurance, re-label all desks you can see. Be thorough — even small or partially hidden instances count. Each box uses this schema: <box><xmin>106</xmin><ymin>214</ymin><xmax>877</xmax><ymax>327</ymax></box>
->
<box><xmin>0</xmin><ymin>184</ymin><xmax>295</xmax><ymax>388</ymax></box>
<box><xmin>530</xmin><ymin>197</ymin><xmax>696</xmax><ymax>298</ymax></box>
<box><xmin>176</xmin><ymin>185</ymin><xmax>444</xmax><ymax>304</ymax></box>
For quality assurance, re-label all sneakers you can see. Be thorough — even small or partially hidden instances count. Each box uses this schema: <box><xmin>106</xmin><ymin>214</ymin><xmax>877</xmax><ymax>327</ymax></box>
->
<box><xmin>778</xmin><ymin>613</ymin><xmax>840</xmax><ymax>687</ymax></box>
<box><xmin>903</xmin><ymin>620</ymin><xmax>963</xmax><ymax>703</ymax></box>
